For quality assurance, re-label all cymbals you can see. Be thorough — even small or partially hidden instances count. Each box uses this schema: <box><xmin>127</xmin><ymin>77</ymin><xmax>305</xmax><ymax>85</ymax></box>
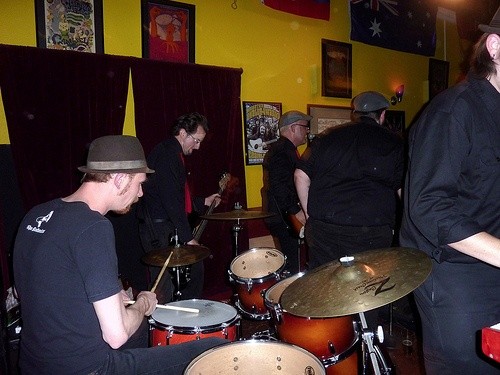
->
<box><xmin>142</xmin><ymin>244</ymin><xmax>210</xmax><ymax>266</ymax></box>
<box><xmin>279</xmin><ymin>247</ymin><xmax>433</xmax><ymax>317</ymax></box>
<box><xmin>199</xmin><ymin>209</ymin><xmax>275</xmax><ymax>222</ymax></box>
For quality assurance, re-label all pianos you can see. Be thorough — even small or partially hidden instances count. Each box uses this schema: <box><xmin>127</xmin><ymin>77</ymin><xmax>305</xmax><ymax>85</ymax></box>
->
<box><xmin>481</xmin><ymin>321</ymin><xmax>500</xmax><ymax>365</ymax></box>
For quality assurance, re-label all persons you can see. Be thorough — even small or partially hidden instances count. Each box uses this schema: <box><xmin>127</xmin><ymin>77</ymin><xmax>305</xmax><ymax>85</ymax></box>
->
<box><xmin>293</xmin><ymin>91</ymin><xmax>408</xmax><ymax>330</ymax></box>
<box><xmin>135</xmin><ymin>112</ymin><xmax>223</xmax><ymax>306</ymax></box>
<box><xmin>263</xmin><ymin>110</ymin><xmax>314</xmax><ymax>275</ymax></box>
<box><xmin>399</xmin><ymin>6</ymin><xmax>500</xmax><ymax>375</ymax></box>
<box><xmin>246</xmin><ymin>116</ymin><xmax>280</xmax><ymax>151</ymax></box>
<box><xmin>12</xmin><ymin>135</ymin><xmax>230</xmax><ymax>375</ymax></box>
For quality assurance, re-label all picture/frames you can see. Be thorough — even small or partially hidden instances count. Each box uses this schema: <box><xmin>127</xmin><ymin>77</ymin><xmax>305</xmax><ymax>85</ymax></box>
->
<box><xmin>34</xmin><ymin>0</ymin><xmax>105</xmax><ymax>54</ymax></box>
<box><xmin>321</xmin><ymin>38</ymin><xmax>352</xmax><ymax>99</ymax></box>
<box><xmin>140</xmin><ymin>0</ymin><xmax>195</xmax><ymax>63</ymax></box>
<box><xmin>306</xmin><ymin>104</ymin><xmax>353</xmax><ymax>151</ymax></box>
<box><xmin>243</xmin><ymin>101</ymin><xmax>282</xmax><ymax>166</ymax></box>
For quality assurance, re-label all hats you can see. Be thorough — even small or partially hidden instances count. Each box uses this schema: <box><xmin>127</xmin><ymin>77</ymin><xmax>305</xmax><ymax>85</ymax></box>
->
<box><xmin>77</xmin><ymin>134</ymin><xmax>156</xmax><ymax>174</ymax></box>
<box><xmin>478</xmin><ymin>6</ymin><xmax>500</xmax><ymax>34</ymax></box>
<box><xmin>278</xmin><ymin>111</ymin><xmax>312</xmax><ymax>129</ymax></box>
<box><xmin>353</xmin><ymin>91</ymin><xmax>390</xmax><ymax>111</ymax></box>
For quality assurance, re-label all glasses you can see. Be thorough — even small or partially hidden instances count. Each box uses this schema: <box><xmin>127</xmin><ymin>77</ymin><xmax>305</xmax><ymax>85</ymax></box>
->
<box><xmin>184</xmin><ymin>132</ymin><xmax>204</xmax><ymax>147</ymax></box>
<box><xmin>291</xmin><ymin>123</ymin><xmax>310</xmax><ymax>132</ymax></box>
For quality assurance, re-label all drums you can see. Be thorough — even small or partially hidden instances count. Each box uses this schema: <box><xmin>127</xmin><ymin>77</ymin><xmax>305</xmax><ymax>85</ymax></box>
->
<box><xmin>147</xmin><ymin>297</ymin><xmax>242</xmax><ymax>348</ymax></box>
<box><xmin>322</xmin><ymin>336</ymin><xmax>364</xmax><ymax>375</ymax></box>
<box><xmin>262</xmin><ymin>271</ymin><xmax>362</xmax><ymax>366</ymax></box>
<box><xmin>227</xmin><ymin>247</ymin><xmax>291</xmax><ymax>320</ymax></box>
<box><xmin>183</xmin><ymin>336</ymin><xmax>326</xmax><ymax>375</ymax></box>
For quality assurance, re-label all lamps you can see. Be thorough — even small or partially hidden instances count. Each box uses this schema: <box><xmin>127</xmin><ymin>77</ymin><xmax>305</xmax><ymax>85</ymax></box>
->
<box><xmin>391</xmin><ymin>85</ymin><xmax>404</xmax><ymax>105</ymax></box>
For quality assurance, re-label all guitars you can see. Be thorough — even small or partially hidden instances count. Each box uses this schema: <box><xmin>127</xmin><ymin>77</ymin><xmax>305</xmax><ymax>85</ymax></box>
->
<box><xmin>166</xmin><ymin>171</ymin><xmax>232</xmax><ymax>299</ymax></box>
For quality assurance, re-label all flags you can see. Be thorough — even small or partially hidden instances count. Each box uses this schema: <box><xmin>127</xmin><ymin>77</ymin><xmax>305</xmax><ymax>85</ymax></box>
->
<box><xmin>350</xmin><ymin>0</ymin><xmax>439</xmax><ymax>58</ymax></box>
